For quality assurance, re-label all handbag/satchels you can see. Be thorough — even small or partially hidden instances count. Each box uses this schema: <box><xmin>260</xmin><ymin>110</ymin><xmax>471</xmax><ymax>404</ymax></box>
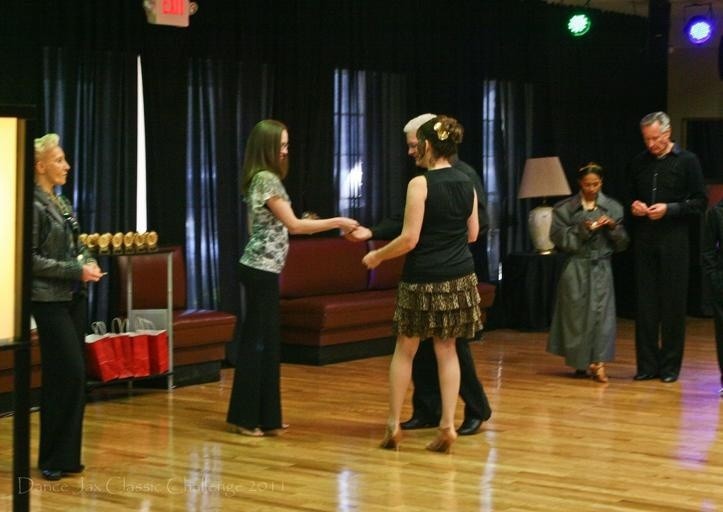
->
<box><xmin>110</xmin><ymin>317</ymin><xmax>136</xmax><ymax>379</ymax></box>
<box><xmin>123</xmin><ymin>317</ymin><xmax>151</xmax><ymax>378</ymax></box>
<box><xmin>135</xmin><ymin>319</ymin><xmax>170</xmax><ymax>377</ymax></box>
<box><xmin>82</xmin><ymin>321</ymin><xmax>120</xmax><ymax>384</ymax></box>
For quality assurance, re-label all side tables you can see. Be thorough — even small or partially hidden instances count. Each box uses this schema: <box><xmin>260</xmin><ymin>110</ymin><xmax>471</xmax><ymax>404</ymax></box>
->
<box><xmin>487</xmin><ymin>252</ymin><xmax>567</xmax><ymax>331</ymax></box>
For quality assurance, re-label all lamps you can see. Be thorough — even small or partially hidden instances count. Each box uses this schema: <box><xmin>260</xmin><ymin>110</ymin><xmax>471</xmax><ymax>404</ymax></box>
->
<box><xmin>518</xmin><ymin>158</ymin><xmax>573</xmax><ymax>255</ymax></box>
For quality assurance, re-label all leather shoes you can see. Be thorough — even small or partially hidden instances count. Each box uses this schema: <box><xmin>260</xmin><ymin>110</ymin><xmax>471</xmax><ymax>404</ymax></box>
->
<box><xmin>59</xmin><ymin>465</ymin><xmax>84</xmax><ymax>472</ymax></box>
<box><xmin>662</xmin><ymin>363</ymin><xmax>681</xmax><ymax>382</ymax></box>
<box><xmin>634</xmin><ymin>371</ymin><xmax>658</xmax><ymax>381</ymax></box>
<box><xmin>401</xmin><ymin>416</ymin><xmax>440</xmax><ymax>428</ymax></box>
<box><xmin>40</xmin><ymin>468</ymin><xmax>62</xmax><ymax>481</ymax></box>
<box><xmin>457</xmin><ymin>408</ymin><xmax>491</xmax><ymax>435</ymax></box>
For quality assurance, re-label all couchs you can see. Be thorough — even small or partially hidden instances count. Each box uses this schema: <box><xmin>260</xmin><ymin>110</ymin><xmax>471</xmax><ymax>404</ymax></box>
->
<box><xmin>278</xmin><ymin>236</ymin><xmax>496</xmax><ymax>366</ymax></box>
<box><xmin>0</xmin><ymin>247</ymin><xmax>236</xmax><ymax>413</ymax></box>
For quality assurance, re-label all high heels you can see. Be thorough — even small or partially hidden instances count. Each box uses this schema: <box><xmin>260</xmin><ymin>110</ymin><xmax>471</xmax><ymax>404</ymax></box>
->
<box><xmin>425</xmin><ymin>426</ymin><xmax>457</xmax><ymax>454</ymax></box>
<box><xmin>380</xmin><ymin>429</ymin><xmax>402</xmax><ymax>452</ymax></box>
<box><xmin>590</xmin><ymin>362</ymin><xmax>608</xmax><ymax>383</ymax></box>
<box><xmin>231</xmin><ymin>419</ymin><xmax>264</xmax><ymax>437</ymax></box>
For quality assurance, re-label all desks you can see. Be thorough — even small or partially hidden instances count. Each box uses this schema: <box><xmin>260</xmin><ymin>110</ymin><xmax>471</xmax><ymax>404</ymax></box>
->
<box><xmin>78</xmin><ymin>245</ymin><xmax>177</xmax><ymax>393</ymax></box>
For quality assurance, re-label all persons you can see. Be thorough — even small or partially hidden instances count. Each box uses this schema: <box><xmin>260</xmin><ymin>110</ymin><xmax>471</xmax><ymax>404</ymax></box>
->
<box><xmin>699</xmin><ymin>197</ymin><xmax>722</xmax><ymax>394</ymax></box>
<box><xmin>31</xmin><ymin>134</ymin><xmax>102</xmax><ymax>480</ymax></box>
<box><xmin>549</xmin><ymin>162</ymin><xmax>628</xmax><ymax>382</ymax></box>
<box><xmin>361</xmin><ymin>116</ymin><xmax>480</xmax><ymax>455</ymax></box>
<box><xmin>622</xmin><ymin>111</ymin><xmax>709</xmax><ymax>385</ymax></box>
<box><xmin>344</xmin><ymin>112</ymin><xmax>495</xmax><ymax>436</ymax></box>
<box><xmin>227</xmin><ymin>118</ymin><xmax>359</xmax><ymax>437</ymax></box>
